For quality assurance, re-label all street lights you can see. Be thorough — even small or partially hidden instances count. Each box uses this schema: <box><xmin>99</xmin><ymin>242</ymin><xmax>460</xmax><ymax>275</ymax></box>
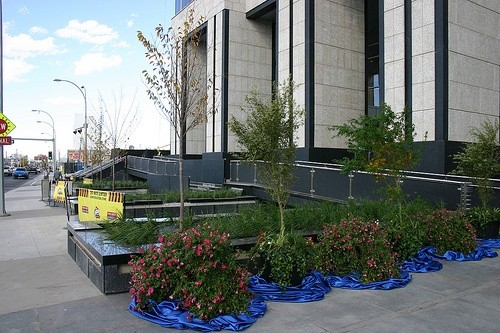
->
<box><xmin>32</xmin><ymin>109</ymin><xmax>57</xmax><ymax>172</ymax></box>
<box><xmin>54</xmin><ymin>78</ymin><xmax>88</xmax><ymax>169</ymax></box>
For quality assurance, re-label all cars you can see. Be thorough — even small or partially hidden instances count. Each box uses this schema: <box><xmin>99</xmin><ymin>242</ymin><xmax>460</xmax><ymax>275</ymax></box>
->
<box><xmin>11</xmin><ymin>163</ymin><xmax>41</xmax><ymax>174</ymax></box>
<box><xmin>13</xmin><ymin>167</ymin><xmax>29</xmax><ymax>179</ymax></box>
<box><xmin>4</xmin><ymin>166</ymin><xmax>12</xmax><ymax>176</ymax></box>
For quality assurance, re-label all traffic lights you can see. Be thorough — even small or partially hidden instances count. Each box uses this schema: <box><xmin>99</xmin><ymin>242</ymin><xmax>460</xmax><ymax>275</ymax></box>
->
<box><xmin>49</xmin><ymin>152</ymin><xmax>52</xmax><ymax>160</ymax></box>
